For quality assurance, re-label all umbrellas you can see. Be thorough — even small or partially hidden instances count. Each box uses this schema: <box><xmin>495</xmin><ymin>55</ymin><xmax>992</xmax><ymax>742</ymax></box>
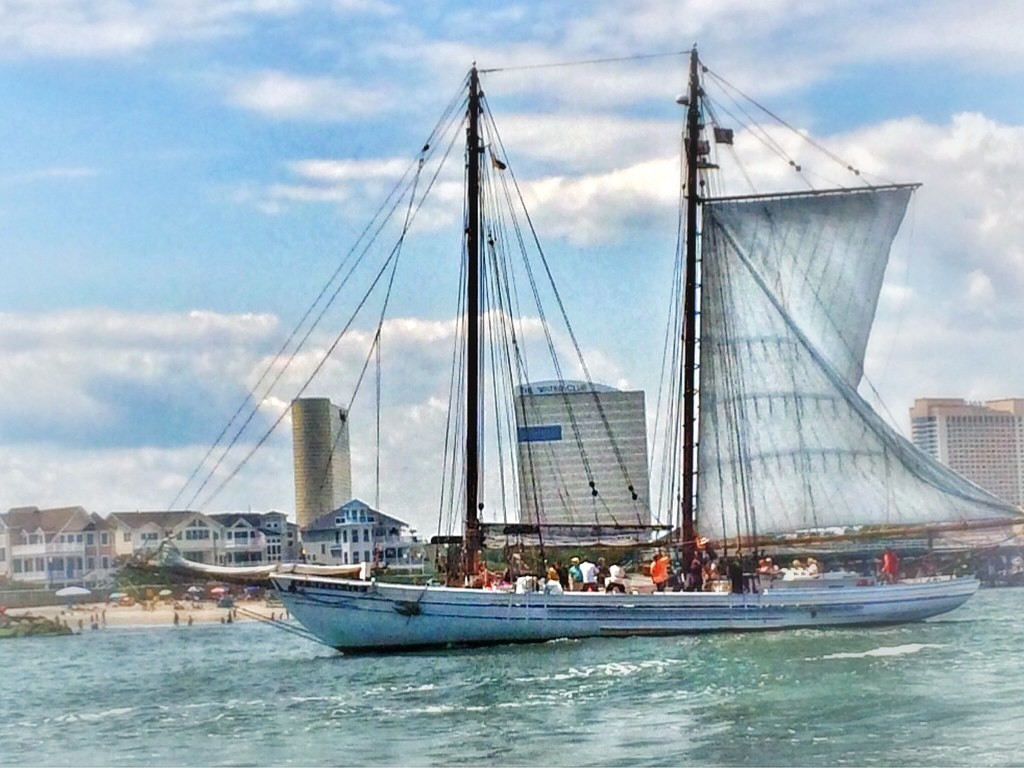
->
<box><xmin>159</xmin><ymin>589</ymin><xmax>172</xmax><ymax>600</ymax></box>
<box><xmin>55</xmin><ymin>586</ymin><xmax>92</xmax><ymax>604</ymax></box>
<box><xmin>210</xmin><ymin>587</ymin><xmax>225</xmax><ymax>596</ymax></box>
<box><xmin>187</xmin><ymin>586</ymin><xmax>203</xmax><ymax>596</ymax></box>
<box><xmin>109</xmin><ymin>592</ymin><xmax>127</xmax><ymax>602</ymax></box>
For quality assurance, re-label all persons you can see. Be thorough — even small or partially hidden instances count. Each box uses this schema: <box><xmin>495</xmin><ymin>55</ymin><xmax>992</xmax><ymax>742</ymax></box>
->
<box><xmin>882</xmin><ymin>548</ymin><xmax>898</xmax><ymax>583</ymax></box>
<box><xmin>544</xmin><ymin>556</ymin><xmax>625</xmax><ymax>593</ymax></box>
<box><xmin>650</xmin><ymin>537</ymin><xmax>820</xmax><ymax>592</ymax></box>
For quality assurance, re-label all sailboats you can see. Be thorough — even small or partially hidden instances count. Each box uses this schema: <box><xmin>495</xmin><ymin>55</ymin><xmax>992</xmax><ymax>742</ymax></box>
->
<box><xmin>110</xmin><ymin>40</ymin><xmax>1024</xmax><ymax>656</ymax></box>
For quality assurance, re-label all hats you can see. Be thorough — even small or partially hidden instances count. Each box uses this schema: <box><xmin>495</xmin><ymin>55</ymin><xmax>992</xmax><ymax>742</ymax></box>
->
<box><xmin>598</xmin><ymin>557</ymin><xmax>606</xmax><ymax>562</ymax></box>
<box><xmin>699</xmin><ymin>537</ymin><xmax>709</xmax><ymax>546</ymax></box>
<box><xmin>570</xmin><ymin>557</ymin><xmax>579</xmax><ymax>563</ymax></box>
<box><xmin>807</xmin><ymin>558</ymin><xmax>815</xmax><ymax>563</ymax></box>
<box><xmin>793</xmin><ymin>559</ymin><xmax>801</xmax><ymax>566</ymax></box>
<box><xmin>765</xmin><ymin>558</ymin><xmax>772</xmax><ymax>563</ymax></box>
